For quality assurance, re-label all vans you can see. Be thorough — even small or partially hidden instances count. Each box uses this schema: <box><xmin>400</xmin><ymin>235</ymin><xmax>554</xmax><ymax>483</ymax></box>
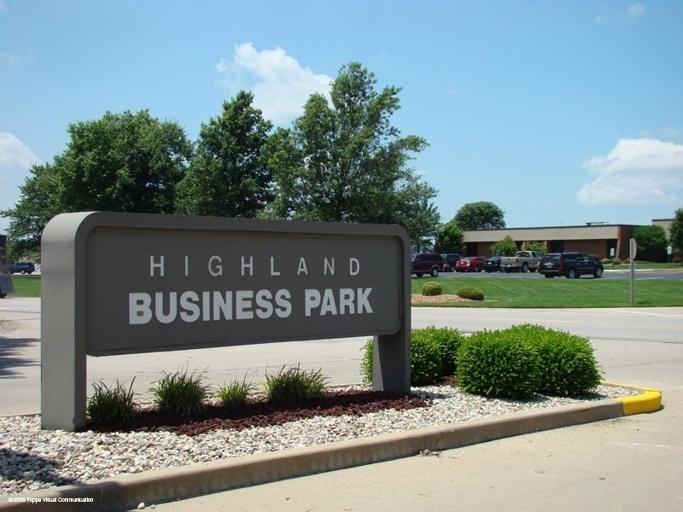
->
<box><xmin>440</xmin><ymin>253</ymin><xmax>462</xmax><ymax>272</ymax></box>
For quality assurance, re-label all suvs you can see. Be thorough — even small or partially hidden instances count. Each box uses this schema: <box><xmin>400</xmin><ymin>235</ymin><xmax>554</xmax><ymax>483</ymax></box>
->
<box><xmin>536</xmin><ymin>251</ymin><xmax>604</xmax><ymax>279</ymax></box>
<box><xmin>410</xmin><ymin>252</ymin><xmax>443</xmax><ymax>277</ymax></box>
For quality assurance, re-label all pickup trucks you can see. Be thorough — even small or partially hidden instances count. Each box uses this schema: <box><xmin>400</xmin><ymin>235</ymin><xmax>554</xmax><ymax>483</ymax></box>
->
<box><xmin>499</xmin><ymin>250</ymin><xmax>542</xmax><ymax>273</ymax></box>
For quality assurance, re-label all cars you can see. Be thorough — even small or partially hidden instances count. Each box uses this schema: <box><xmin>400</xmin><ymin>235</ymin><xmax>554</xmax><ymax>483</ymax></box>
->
<box><xmin>483</xmin><ymin>255</ymin><xmax>503</xmax><ymax>273</ymax></box>
<box><xmin>8</xmin><ymin>261</ymin><xmax>35</xmax><ymax>274</ymax></box>
<box><xmin>455</xmin><ymin>256</ymin><xmax>486</xmax><ymax>272</ymax></box>
